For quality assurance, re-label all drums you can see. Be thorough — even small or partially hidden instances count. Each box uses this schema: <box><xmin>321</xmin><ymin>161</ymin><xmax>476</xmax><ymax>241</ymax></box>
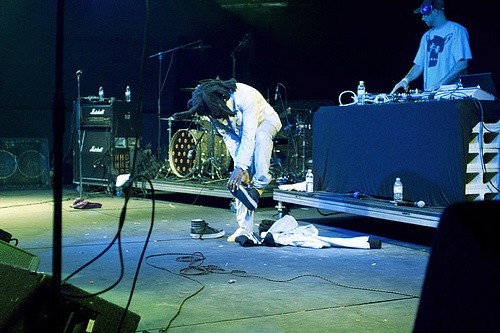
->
<box><xmin>294</xmin><ymin>121</ymin><xmax>312</xmax><ymax>137</ymax></box>
<box><xmin>199</xmin><ymin>116</ymin><xmax>216</xmax><ymax>132</ymax></box>
<box><xmin>167</xmin><ymin>128</ymin><xmax>230</xmax><ymax>178</ymax></box>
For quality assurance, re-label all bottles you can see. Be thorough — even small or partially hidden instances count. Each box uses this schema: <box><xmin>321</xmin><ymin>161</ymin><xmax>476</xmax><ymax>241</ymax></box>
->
<box><xmin>125</xmin><ymin>86</ymin><xmax>131</xmax><ymax>102</ymax></box>
<box><xmin>357</xmin><ymin>81</ymin><xmax>365</xmax><ymax>105</ymax></box>
<box><xmin>393</xmin><ymin>178</ymin><xmax>403</xmax><ymax>206</ymax></box>
<box><xmin>305</xmin><ymin>169</ymin><xmax>313</xmax><ymax>193</ymax></box>
<box><xmin>99</xmin><ymin>86</ymin><xmax>104</xmax><ymax>101</ymax></box>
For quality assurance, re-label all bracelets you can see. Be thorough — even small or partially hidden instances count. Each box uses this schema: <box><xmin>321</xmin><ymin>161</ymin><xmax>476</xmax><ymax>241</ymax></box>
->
<box><xmin>402</xmin><ymin>78</ymin><xmax>408</xmax><ymax>84</ymax></box>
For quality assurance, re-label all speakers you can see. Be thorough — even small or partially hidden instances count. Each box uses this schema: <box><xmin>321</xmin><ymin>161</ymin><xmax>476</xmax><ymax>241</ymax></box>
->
<box><xmin>1</xmin><ymin>138</ymin><xmax>52</xmax><ymax>191</ymax></box>
<box><xmin>76</xmin><ymin>125</ymin><xmax>112</xmax><ymax>179</ymax></box>
<box><xmin>409</xmin><ymin>199</ymin><xmax>500</xmax><ymax>333</ymax></box>
<box><xmin>0</xmin><ymin>230</ymin><xmax>141</xmax><ymax>333</ymax></box>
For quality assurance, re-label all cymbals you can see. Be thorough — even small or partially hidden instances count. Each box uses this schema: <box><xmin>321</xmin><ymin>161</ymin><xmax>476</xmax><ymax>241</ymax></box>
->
<box><xmin>179</xmin><ymin>86</ymin><xmax>200</xmax><ymax>92</ymax></box>
<box><xmin>199</xmin><ymin>79</ymin><xmax>224</xmax><ymax>83</ymax></box>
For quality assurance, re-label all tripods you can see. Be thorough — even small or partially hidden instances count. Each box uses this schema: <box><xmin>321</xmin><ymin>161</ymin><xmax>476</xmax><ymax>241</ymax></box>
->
<box><xmin>144</xmin><ymin>41</ymin><xmax>312</xmax><ymax>183</ymax></box>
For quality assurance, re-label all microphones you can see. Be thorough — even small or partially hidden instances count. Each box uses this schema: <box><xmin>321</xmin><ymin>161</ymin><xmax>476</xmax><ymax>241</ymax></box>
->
<box><xmin>395</xmin><ymin>199</ymin><xmax>426</xmax><ymax>208</ymax></box>
<box><xmin>353</xmin><ymin>192</ymin><xmax>366</xmax><ymax>198</ymax></box>
<box><xmin>275</xmin><ymin>85</ymin><xmax>279</xmax><ymax>99</ymax></box>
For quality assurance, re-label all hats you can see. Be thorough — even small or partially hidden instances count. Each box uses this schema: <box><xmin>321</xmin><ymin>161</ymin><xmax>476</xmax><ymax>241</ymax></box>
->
<box><xmin>413</xmin><ymin>0</ymin><xmax>445</xmax><ymax>13</ymax></box>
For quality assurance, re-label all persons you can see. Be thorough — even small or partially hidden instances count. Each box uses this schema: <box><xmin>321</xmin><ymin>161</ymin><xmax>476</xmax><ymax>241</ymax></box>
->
<box><xmin>390</xmin><ymin>0</ymin><xmax>473</xmax><ymax>94</ymax></box>
<box><xmin>187</xmin><ymin>79</ymin><xmax>282</xmax><ymax>242</ymax></box>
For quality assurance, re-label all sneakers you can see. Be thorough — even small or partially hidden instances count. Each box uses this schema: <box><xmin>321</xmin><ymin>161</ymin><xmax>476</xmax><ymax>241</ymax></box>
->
<box><xmin>190</xmin><ymin>219</ymin><xmax>226</xmax><ymax>240</ymax></box>
<box><xmin>229</xmin><ymin>185</ymin><xmax>260</xmax><ymax>211</ymax></box>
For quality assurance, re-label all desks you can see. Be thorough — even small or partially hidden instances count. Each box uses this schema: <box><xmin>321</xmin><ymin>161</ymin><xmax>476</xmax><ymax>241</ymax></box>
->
<box><xmin>312</xmin><ymin>100</ymin><xmax>484</xmax><ymax>205</ymax></box>
